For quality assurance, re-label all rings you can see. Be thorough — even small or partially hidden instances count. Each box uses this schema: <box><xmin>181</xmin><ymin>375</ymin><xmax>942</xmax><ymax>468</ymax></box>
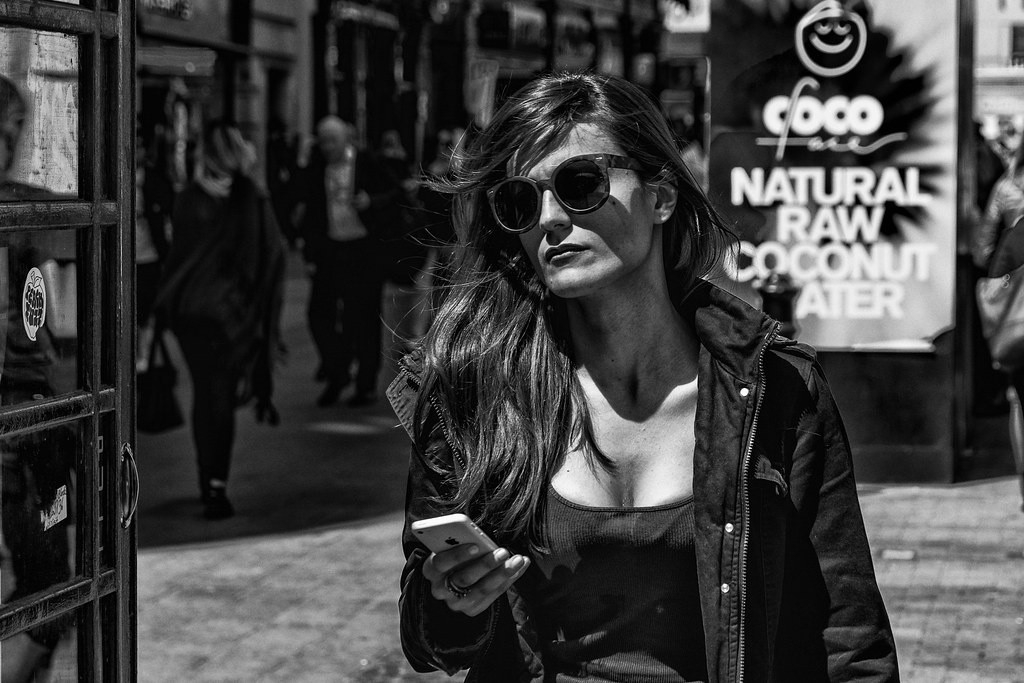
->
<box><xmin>445</xmin><ymin>574</ymin><xmax>474</xmax><ymax>598</ymax></box>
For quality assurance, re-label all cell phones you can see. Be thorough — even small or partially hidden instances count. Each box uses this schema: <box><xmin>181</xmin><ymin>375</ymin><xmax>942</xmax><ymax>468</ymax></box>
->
<box><xmin>412</xmin><ymin>514</ymin><xmax>500</xmax><ymax>560</ymax></box>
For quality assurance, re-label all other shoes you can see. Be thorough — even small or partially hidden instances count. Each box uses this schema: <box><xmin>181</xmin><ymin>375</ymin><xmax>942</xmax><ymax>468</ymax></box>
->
<box><xmin>202</xmin><ymin>481</ymin><xmax>233</xmax><ymax>520</ymax></box>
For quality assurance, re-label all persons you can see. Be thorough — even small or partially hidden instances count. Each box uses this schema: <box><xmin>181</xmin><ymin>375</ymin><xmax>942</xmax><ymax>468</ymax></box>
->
<box><xmin>157</xmin><ymin>114</ymin><xmax>471</xmax><ymax>520</ymax></box>
<box><xmin>386</xmin><ymin>73</ymin><xmax>902</xmax><ymax>683</ymax></box>
<box><xmin>978</xmin><ymin>115</ymin><xmax>1024</xmax><ymax>511</ymax></box>
<box><xmin>0</xmin><ymin>76</ymin><xmax>96</xmax><ymax>682</ymax></box>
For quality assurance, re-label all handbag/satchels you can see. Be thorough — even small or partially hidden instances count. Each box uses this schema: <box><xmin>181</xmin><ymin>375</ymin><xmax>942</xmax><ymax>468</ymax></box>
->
<box><xmin>136</xmin><ymin>367</ymin><xmax>187</xmax><ymax>435</ymax></box>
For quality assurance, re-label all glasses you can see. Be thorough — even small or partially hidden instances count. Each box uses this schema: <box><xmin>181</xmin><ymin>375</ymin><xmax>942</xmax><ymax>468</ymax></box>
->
<box><xmin>482</xmin><ymin>152</ymin><xmax>646</xmax><ymax>235</ymax></box>
